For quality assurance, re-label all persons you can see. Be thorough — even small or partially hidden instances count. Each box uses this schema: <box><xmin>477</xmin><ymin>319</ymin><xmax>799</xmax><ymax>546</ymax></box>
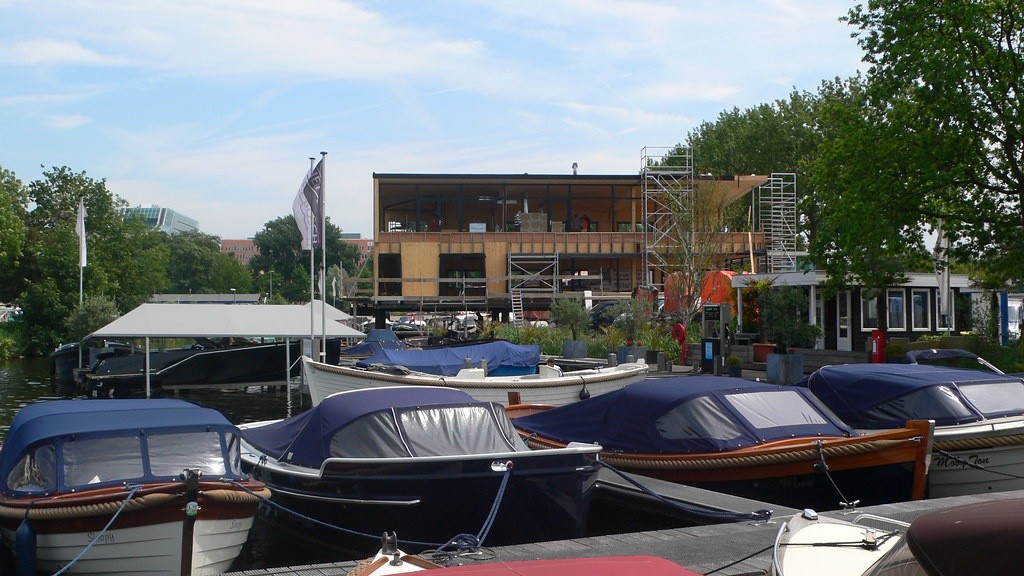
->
<box><xmin>570</xmin><ymin>214</ymin><xmax>591</xmax><ymax>231</ymax></box>
<box><xmin>515</xmin><ymin>210</ymin><xmax>522</xmax><ymax>232</ymax></box>
<box><xmin>724</xmin><ymin>323</ymin><xmax>731</xmax><ymax>342</ymax></box>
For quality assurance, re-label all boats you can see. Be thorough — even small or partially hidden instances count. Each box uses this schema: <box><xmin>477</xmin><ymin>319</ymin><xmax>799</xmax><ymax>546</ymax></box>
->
<box><xmin>52</xmin><ymin>339</ymin><xmax>342</xmax><ymax>400</ymax></box>
<box><xmin>773</xmin><ymin>498</ymin><xmax>1024</xmax><ymax>576</ymax></box>
<box><xmin>361</xmin><ymin>330</ymin><xmax>540</xmax><ymax>374</ymax></box>
<box><xmin>299</xmin><ymin>353</ymin><xmax>649</xmax><ymax>409</ymax></box>
<box><xmin>364</xmin><ymin>312</ymin><xmax>490</xmax><ymax>329</ymax></box>
<box><xmin>502</xmin><ymin>377</ymin><xmax>935</xmax><ymax>511</ymax></box>
<box><xmin>0</xmin><ymin>399</ymin><xmax>269</xmax><ymax>576</ymax></box>
<box><xmin>230</xmin><ymin>387</ymin><xmax>604</xmax><ymax>550</ymax></box>
<box><xmin>798</xmin><ymin>347</ymin><xmax>1023</xmax><ymax>497</ymax></box>
<box><xmin>354</xmin><ymin>531</ymin><xmax>701</xmax><ymax>576</ymax></box>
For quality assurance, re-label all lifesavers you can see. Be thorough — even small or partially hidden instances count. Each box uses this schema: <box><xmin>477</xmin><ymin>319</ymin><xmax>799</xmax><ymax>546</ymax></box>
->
<box><xmin>672</xmin><ymin>323</ymin><xmax>685</xmax><ymax>343</ymax></box>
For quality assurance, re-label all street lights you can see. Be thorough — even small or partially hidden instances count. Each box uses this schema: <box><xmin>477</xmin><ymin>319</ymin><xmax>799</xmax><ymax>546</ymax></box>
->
<box><xmin>268</xmin><ymin>270</ymin><xmax>274</xmax><ymax>299</ymax></box>
<box><xmin>231</xmin><ymin>289</ymin><xmax>235</xmax><ymax>302</ymax></box>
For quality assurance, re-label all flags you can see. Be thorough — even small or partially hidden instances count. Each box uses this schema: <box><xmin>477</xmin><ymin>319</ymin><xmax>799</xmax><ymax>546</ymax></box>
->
<box><xmin>292</xmin><ymin>158</ymin><xmax>324</xmax><ymax>249</ymax></box>
<box><xmin>75</xmin><ymin>201</ymin><xmax>88</xmax><ymax>268</ymax></box>
<box><xmin>932</xmin><ymin>210</ymin><xmax>951</xmax><ymax>315</ymax></box>
